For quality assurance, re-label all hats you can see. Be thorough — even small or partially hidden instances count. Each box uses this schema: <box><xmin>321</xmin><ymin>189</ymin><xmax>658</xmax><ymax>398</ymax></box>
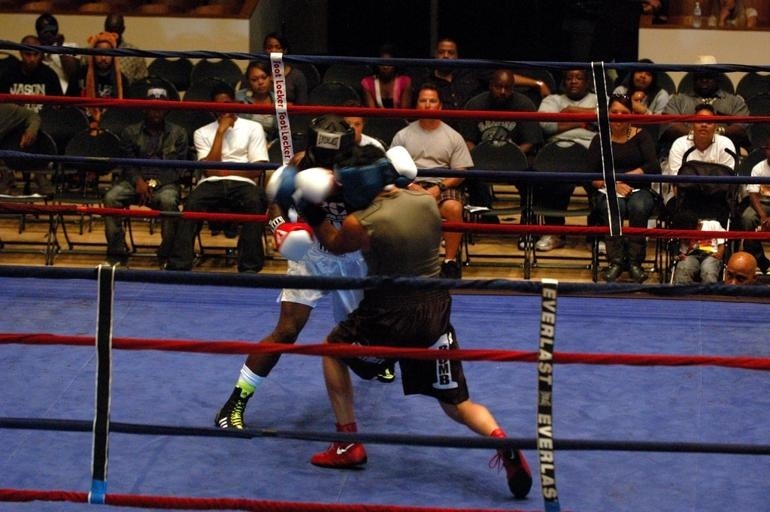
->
<box><xmin>86</xmin><ymin>31</ymin><xmax>120</xmax><ymax>63</ymax></box>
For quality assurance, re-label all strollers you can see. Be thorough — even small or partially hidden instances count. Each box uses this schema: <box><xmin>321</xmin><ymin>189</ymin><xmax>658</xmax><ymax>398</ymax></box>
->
<box><xmin>653</xmin><ymin>144</ymin><xmax>743</xmax><ymax>286</ymax></box>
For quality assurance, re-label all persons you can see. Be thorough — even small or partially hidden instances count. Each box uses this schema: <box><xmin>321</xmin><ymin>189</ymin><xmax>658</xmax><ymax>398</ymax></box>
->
<box><xmin>0</xmin><ymin>2</ymin><xmax>767</xmax><ymax>284</ymax></box>
<box><xmin>292</xmin><ymin>143</ymin><xmax>532</xmax><ymax>502</ymax></box>
<box><xmin>214</xmin><ymin>101</ymin><xmax>396</xmax><ymax>430</ymax></box>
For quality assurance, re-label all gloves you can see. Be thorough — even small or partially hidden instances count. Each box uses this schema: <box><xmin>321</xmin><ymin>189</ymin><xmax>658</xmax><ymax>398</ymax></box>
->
<box><xmin>266</xmin><ymin>164</ymin><xmax>332</xmax><ymax>262</ymax></box>
<box><xmin>385</xmin><ymin>145</ymin><xmax>418</xmax><ymax>187</ymax></box>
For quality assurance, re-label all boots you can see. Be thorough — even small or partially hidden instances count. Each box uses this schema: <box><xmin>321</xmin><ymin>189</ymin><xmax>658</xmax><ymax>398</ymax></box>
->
<box><xmin>590</xmin><ymin>234</ymin><xmax>648</xmax><ymax>282</ymax></box>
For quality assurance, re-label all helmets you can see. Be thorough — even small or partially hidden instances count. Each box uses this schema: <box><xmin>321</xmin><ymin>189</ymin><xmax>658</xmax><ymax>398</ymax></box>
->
<box><xmin>334</xmin><ymin>157</ymin><xmax>397</xmax><ymax>209</ymax></box>
<box><xmin>307</xmin><ymin>118</ymin><xmax>356</xmax><ymax>164</ymax></box>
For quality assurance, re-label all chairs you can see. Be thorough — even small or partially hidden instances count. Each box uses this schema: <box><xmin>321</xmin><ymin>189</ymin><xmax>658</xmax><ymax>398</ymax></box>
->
<box><xmin>0</xmin><ymin>55</ymin><xmax>770</xmax><ymax>287</ymax></box>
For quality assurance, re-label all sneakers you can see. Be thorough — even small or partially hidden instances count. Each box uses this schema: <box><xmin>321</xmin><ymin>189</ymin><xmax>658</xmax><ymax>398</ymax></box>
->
<box><xmin>518</xmin><ymin>234</ymin><xmax>565</xmax><ymax>251</ymax></box>
<box><xmin>214</xmin><ymin>381</ymin><xmax>254</xmax><ymax>429</ymax></box>
<box><xmin>501</xmin><ymin>446</ymin><xmax>532</xmax><ymax>499</ymax></box>
<box><xmin>312</xmin><ymin>439</ymin><xmax>368</xmax><ymax>468</ymax></box>
<box><xmin>442</xmin><ymin>259</ymin><xmax>462</xmax><ymax>279</ymax></box>
